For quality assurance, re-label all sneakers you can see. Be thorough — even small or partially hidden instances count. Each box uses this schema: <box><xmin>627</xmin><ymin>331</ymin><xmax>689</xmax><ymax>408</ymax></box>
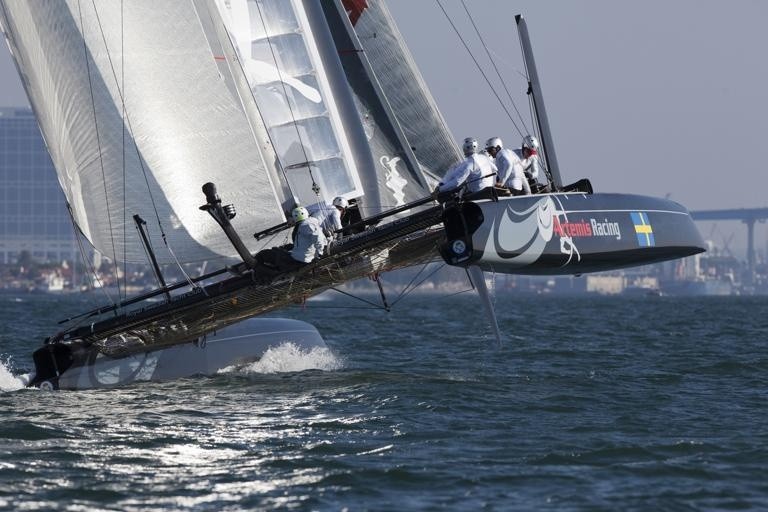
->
<box><xmin>225</xmin><ymin>265</ymin><xmax>240</xmax><ymax>275</ymax></box>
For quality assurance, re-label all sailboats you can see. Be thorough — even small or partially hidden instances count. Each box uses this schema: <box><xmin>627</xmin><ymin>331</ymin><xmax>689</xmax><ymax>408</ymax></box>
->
<box><xmin>0</xmin><ymin>1</ymin><xmax>717</xmax><ymax>393</ymax></box>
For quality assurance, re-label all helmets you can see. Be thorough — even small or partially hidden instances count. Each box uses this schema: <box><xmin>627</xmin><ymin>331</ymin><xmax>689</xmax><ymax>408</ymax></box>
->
<box><xmin>463</xmin><ymin>137</ymin><xmax>503</xmax><ymax>163</ymax></box>
<box><xmin>522</xmin><ymin>135</ymin><xmax>537</xmax><ymax>151</ymax></box>
<box><xmin>333</xmin><ymin>197</ymin><xmax>349</xmax><ymax>209</ymax></box>
<box><xmin>292</xmin><ymin>207</ymin><xmax>308</xmax><ymax>224</ymax></box>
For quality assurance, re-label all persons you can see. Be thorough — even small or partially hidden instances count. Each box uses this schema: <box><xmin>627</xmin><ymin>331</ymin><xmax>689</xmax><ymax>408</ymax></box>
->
<box><xmin>224</xmin><ymin>206</ymin><xmax>327</xmax><ymax>279</ymax></box>
<box><xmin>432</xmin><ymin>135</ymin><xmax>548</xmax><ymax>204</ymax></box>
<box><xmin>313</xmin><ymin>197</ymin><xmax>354</xmax><ymax>242</ymax></box>
